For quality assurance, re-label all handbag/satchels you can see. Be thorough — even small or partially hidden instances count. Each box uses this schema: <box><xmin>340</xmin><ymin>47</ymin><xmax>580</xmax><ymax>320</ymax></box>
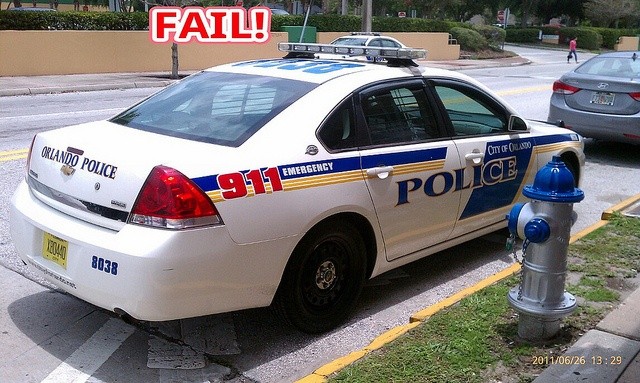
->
<box><xmin>567</xmin><ymin>55</ymin><xmax>573</xmax><ymax>58</ymax></box>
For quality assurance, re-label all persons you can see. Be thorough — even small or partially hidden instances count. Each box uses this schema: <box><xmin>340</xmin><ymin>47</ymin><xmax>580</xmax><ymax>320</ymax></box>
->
<box><xmin>567</xmin><ymin>36</ymin><xmax>579</xmax><ymax>63</ymax></box>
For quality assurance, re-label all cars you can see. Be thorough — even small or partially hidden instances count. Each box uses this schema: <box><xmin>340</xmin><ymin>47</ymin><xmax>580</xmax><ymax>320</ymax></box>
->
<box><xmin>548</xmin><ymin>50</ymin><xmax>640</xmax><ymax>149</ymax></box>
<box><xmin>8</xmin><ymin>32</ymin><xmax>586</xmax><ymax>333</ymax></box>
<box><xmin>318</xmin><ymin>32</ymin><xmax>427</xmax><ymax>64</ymax></box>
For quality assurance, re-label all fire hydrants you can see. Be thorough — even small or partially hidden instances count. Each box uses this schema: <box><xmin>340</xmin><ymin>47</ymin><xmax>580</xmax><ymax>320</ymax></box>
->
<box><xmin>506</xmin><ymin>154</ymin><xmax>584</xmax><ymax>342</ymax></box>
